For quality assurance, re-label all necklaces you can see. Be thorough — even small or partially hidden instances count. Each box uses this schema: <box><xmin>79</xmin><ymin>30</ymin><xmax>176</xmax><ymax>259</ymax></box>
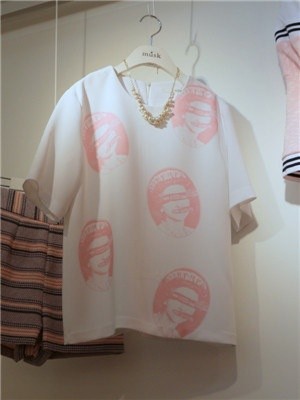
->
<box><xmin>123</xmin><ymin>57</ymin><xmax>182</xmax><ymax>128</ymax></box>
